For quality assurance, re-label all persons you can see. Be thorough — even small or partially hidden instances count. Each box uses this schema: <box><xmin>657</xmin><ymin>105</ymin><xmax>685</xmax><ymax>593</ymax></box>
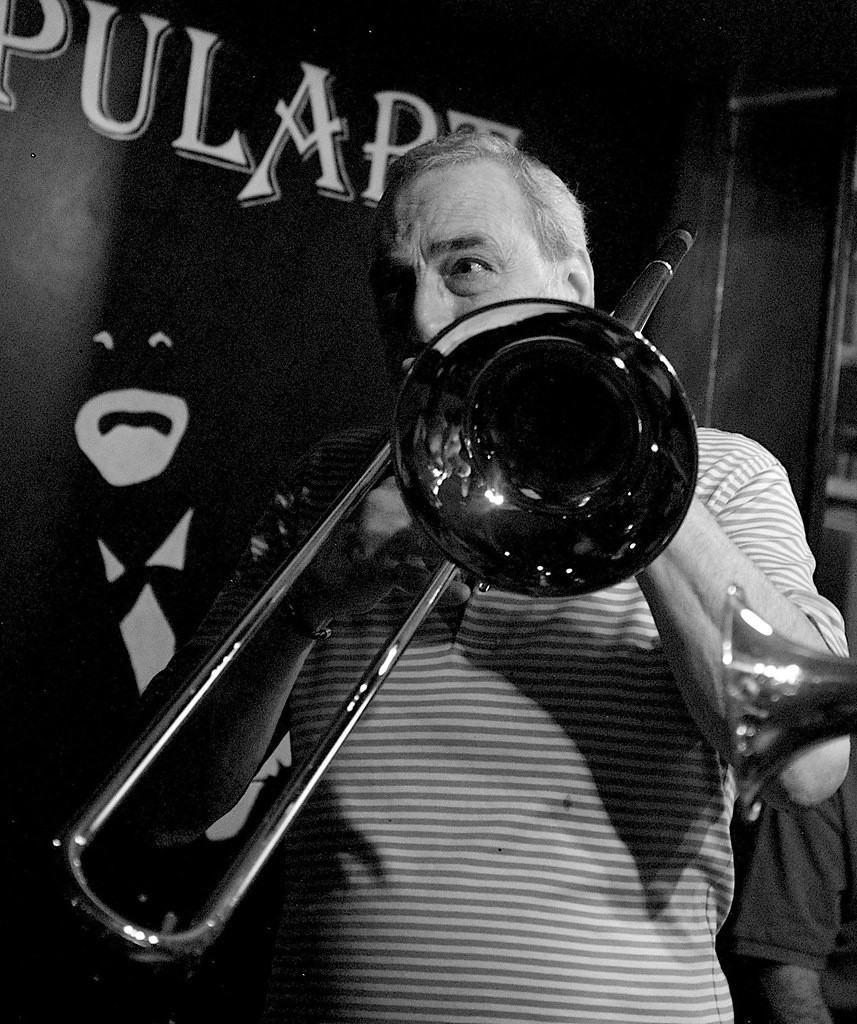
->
<box><xmin>735</xmin><ymin>740</ymin><xmax>857</xmax><ymax>1024</ymax></box>
<box><xmin>108</xmin><ymin>131</ymin><xmax>854</xmax><ymax>1023</ymax></box>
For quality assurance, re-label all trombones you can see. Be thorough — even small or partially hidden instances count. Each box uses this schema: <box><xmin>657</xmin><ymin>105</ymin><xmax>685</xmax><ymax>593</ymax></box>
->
<box><xmin>58</xmin><ymin>211</ymin><xmax>707</xmax><ymax>978</ymax></box>
